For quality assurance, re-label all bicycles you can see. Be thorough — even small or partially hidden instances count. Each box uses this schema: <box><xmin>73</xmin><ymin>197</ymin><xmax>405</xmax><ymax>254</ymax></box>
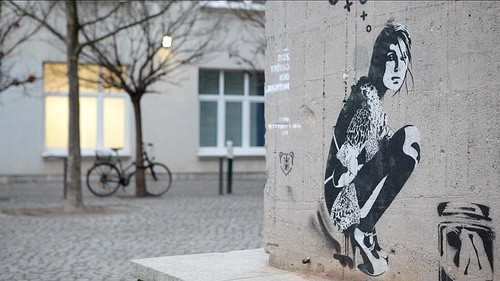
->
<box><xmin>86</xmin><ymin>141</ymin><xmax>173</xmax><ymax>197</ymax></box>
